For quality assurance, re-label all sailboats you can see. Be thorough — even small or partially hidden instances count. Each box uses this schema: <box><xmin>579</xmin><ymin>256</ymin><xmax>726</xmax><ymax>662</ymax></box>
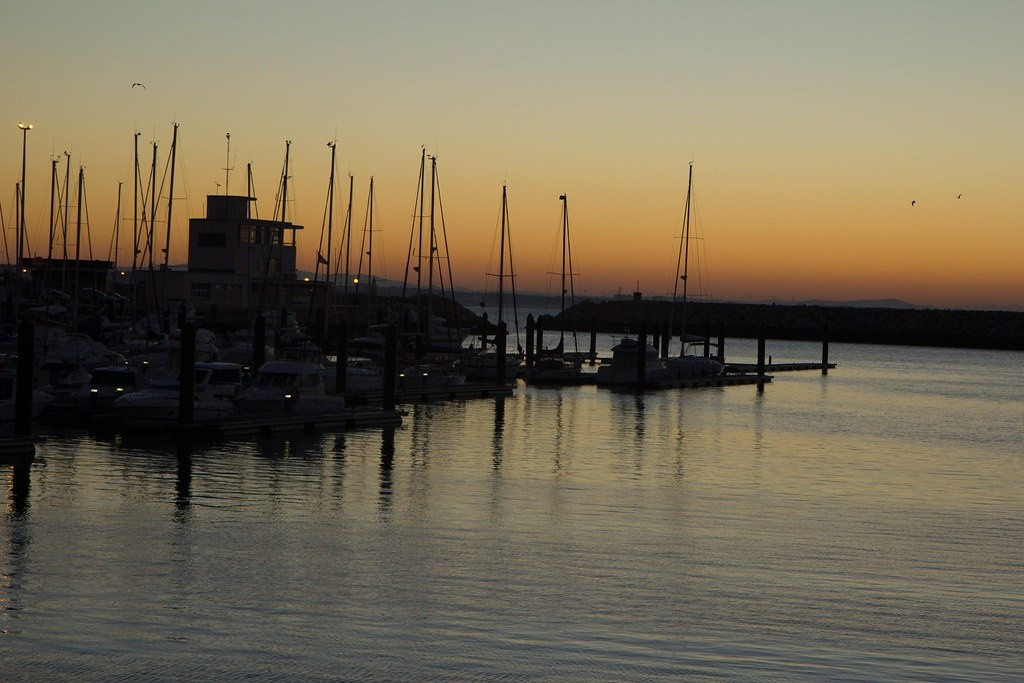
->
<box><xmin>0</xmin><ymin>120</ymin><xmax>727</xmax><ymax>442</ymax></box>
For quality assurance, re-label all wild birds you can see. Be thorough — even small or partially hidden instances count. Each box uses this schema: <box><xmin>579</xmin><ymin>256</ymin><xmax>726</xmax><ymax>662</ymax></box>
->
<box><xmin>131</xmin><ymin>83</ymin><xmax>146</xmax><ymax>90</ymax></box>
<box><xmin>957</xmin><ymin>194</ymin><xmax>962</xmax><ymax>199</ymax></box>
<box><xmin>912</xmin><ymin>200</ymin><xmax>916</xmax><ymax>206</ymax></box>
